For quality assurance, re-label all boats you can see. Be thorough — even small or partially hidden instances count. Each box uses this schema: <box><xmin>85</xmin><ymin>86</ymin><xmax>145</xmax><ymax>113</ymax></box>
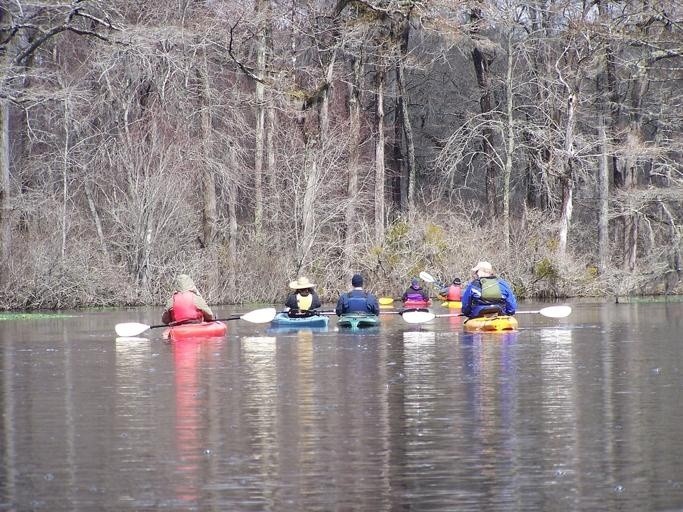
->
<box><xmin>271</xmin><ymin>310</ymin><xmax>329</xmax><ymax>329</ymax></box>
<box><xmin>403</xmin><ymin>300</ymin><xmax>432</xmax><ymax>308</ymax></box>
<box><xmin>462</xmin><ymin>314</ymin><xmax>519</xmax><ymax>334</ymax></box>
<box><xmin>335</xmin><ymin>312</ymin><xmax>382</xmax><ymax>329</ymax></box>
<box><xmin>167</xmin><ymin>320</ymin><xmax>228</xmax><ymax>340</ymax></box>
<box><xmin>441</xmin><ymin>300</ymin><xmax>462</xmax><ymax>309</ymax></box>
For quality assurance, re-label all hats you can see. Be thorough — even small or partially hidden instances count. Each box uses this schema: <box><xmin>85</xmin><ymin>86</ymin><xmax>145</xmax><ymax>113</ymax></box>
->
<box><xmin>353</xmin><ymin>275</ymin><xmax>361</xmax><ymax>285</ymax></box>
<box><xmin>472</xmin><ymin>262</ymin><xmax>492</xmax><ymax>271</ymax></box>
<box><xmin>288</xmin><ymin>277</ymin><xmax>316</xmax><ymax>289</ymax></box>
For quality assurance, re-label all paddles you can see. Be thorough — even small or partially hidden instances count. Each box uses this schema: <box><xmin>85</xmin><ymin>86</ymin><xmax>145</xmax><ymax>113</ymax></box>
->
<box><xmin>402</xmin><ymin>306</ymin><xmax>572</xmax><ymax>324</ymax></box>
<box><xmin>379</xmin><ymin>297</ymin><xmax>402</xmax><ymax>305</ymax></box>
<box><xmin>115</xmin><ymin>307</ymin><xmax>276</xmax><ymax>336</ymax></box>
<box><xmin>289</xmin><ymin>308</ymin><xmax>429</xmax><ymax>318</ymax></box>
<box><xmin>420</xmin><ymin>271</ymin><xmax>444</xmax><ymax>287</ymax></box>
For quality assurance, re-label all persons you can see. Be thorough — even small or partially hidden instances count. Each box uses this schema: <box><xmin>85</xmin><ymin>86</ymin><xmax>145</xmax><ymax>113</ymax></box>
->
<box><xmin>438</xmin><ymin>277</ymin><xmax>466</xmax><ymax>302</ymax></box>
<box><xmin>460</xmin><ymin>260</ymin><xmax>516</xmax><ymax>317</ymax></box>
<box><xmin>158</xmin><ymin>274</ymin><xmax>213</xmax><ymax>322</ymax></box>
<box><xmin>335</xmin><ymin>274</ymin><xmax>380</xmax><ymax>315</ymax></box>
<box><xmin>282</xmin><ymin>276</ymin><xmax>320</xmax><ymax>315</ymax></box>
<box><xmin>399</xmin><ymin>279</ymin><xmax>429</xmax><ymax>303</ymax></box>
